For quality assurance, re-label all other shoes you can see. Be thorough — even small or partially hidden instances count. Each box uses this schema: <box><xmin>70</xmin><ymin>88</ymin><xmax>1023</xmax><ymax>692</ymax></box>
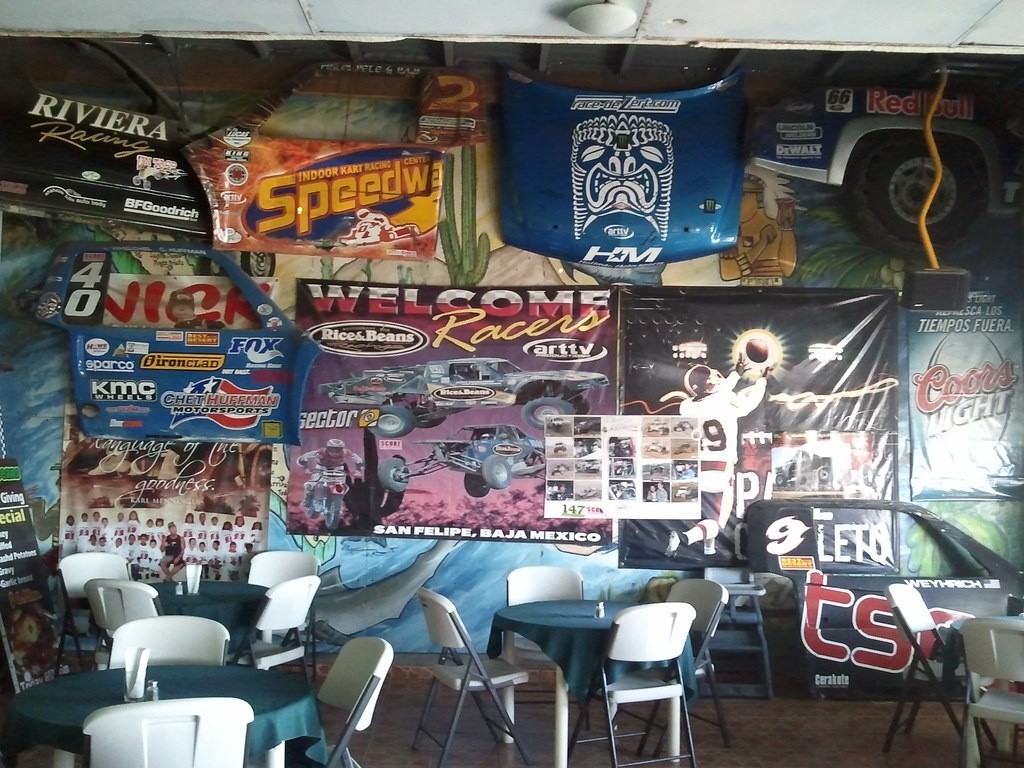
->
<box><xmin>301</xmin><ymin>491</ymin><xmax>312</xmax><ymax>507</ymax></box>
<box><xmin>704</xmin><ymin>549</ymin><xmax>732</xmax><ymax>561</ymax></box>
<box><xmin>664</xmin><ymin>531</ymin><xmax>685</xmax><ymax>563</ymax></box>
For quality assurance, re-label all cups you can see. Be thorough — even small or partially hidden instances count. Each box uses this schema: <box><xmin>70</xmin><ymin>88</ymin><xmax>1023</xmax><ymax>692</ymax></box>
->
<box><xmin>185</xmin><ymin>565</ymin><xmax>202</xmax><ymax>596</ymax></box>
<box><xmin>124</xmin><ymin>648</ymin><xmax>150</xmax><ymax>702</ymax></box>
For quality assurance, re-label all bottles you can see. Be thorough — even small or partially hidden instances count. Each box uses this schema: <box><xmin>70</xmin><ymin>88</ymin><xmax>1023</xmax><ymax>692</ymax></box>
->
<box><xmin>175</xmin><ymin>582</ymin><xmax>183</xmax><ymax>595</ymax></box>
<box><xmin>146</xmin><ymin>680</ymin><xmax>159</xmax><ymax>700</ymax></box>
<box><xmin>595</xmin><ymin>601</ymin><xmax>605</xmax><ymax>619</ymax></box>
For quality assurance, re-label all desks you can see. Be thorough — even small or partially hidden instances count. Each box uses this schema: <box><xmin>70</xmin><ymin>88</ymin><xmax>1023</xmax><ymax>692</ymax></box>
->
<box><xmin>2</xmin><ymin>666</ymin><xmax>330</xmax><ymax>768</ymax></box>
<box><xmin>144</xmin><ymin>582</ymin><xmax>269</xmax><ymax>655</ymax></box>
<box><xmin>943</xmin><ymin>615</ymin><xmax>1024</xmax><ymax>751</ymax></box>
<box><xmin>486</xmin><ymin>601</ymin><xmax>699</xmax><ymax>767</ymax></box>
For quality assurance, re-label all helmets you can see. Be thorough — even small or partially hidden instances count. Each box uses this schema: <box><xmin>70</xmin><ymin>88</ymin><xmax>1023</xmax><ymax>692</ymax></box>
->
<box><xmin>327</xmin><ymin>439</ymin><xmax>345</xmax><ymax>447</ymax></box>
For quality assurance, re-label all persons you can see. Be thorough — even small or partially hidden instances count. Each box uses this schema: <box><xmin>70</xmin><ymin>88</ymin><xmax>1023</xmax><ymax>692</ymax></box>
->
<box><xmin>62</xmin><ymin>508</ymin><xmax>265</xmax><ymax>584</ymax></box>
<box><xmin>664</xmin><ymin>352</ymin><xmax>776</xmax><ymax>558</ymax></box>
<box><xmin>296</xmin><ymin>439</ymin><xmax>363</xmax><ymax>509</ymax></box>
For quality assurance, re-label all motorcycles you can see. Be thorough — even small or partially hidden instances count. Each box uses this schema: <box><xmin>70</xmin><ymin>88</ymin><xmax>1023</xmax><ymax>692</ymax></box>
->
<box><xmin>299</xmin><ymin>460</ymin><xmax>361</xmax><ymax>530</ymax></box>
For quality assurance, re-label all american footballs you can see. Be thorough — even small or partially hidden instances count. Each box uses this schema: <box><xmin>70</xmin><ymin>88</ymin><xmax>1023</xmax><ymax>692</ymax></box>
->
<box><xmin>746</xmin><ymin>338</ymin><xmax>768</xmax><ymax>363</ymax></box>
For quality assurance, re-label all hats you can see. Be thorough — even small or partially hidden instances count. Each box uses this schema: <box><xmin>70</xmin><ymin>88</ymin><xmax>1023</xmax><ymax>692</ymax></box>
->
<box><xmin>689</xmin><ymin>366</ymin><xmax>711</xmax><ymax>386</ymax></box>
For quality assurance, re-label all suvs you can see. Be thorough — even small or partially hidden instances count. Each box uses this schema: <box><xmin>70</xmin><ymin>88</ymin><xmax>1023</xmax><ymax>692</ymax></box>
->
<box><xmin>377</xmin><ymin>422</ymin><xmax>546</xmax><ymax>498</ymax></box>
<box><xmin>776</xmin><ymin>450</ymin><xmax>834</xmax><ymax>488</ymax></box>
<box><xmin>319</xmin><ymin>357</ymin><xmax>611</xmax><ymax>438</ymax></box>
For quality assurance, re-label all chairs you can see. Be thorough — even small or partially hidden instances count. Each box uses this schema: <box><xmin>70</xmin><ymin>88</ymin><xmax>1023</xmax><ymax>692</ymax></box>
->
<box><xmin>52</xmin><ymin>549</ymin><xmax>394</xmax><ymax>768</ymax></box>
<box><xmin>636</xmin><ymin>578</ymin><xmax>730</xmax><ymax>757</ymax></box>
<box><xmin>502</xmin><ymin>566</ymin><xmax>584</xmax><ymax>743</ymax></box>
<box><xmin>411</xmin><ymin>587</ymin><xmax>533</xmax><ymax>768</ymax></box>
<box><xmin>568</xmin><ymin>602</ymin><xmax>697</xmax><ymax>768</ymax></box>
<box><xmin>883</xmin><ymin>584</ymin><xmax>1024</xmax><ymax>768</ymax></box>
